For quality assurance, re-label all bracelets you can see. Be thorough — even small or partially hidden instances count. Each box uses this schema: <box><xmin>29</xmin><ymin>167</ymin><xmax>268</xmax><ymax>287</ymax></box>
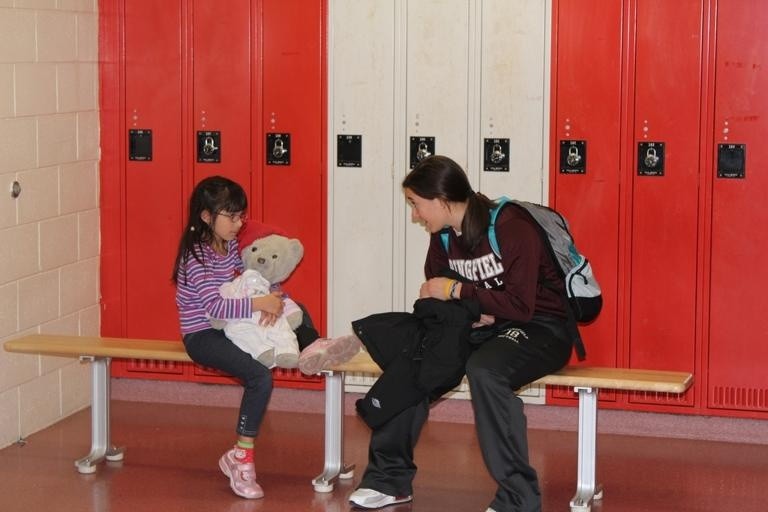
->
<box><xmin>444</xmin><ymin>279</ymin><xmax>456</xmax><ymax>301</ymax></box>
<box><xmin>450</xmin><ymin>281</ymin><xmax>459</xmax><ymax>299</ymax></box>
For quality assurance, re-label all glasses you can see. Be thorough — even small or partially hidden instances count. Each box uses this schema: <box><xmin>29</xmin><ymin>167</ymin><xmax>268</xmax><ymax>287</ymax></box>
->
<box><xmin>216</xmin><ymin>214</ymin><xmax>247</xmax><ymax>223</ymax></box>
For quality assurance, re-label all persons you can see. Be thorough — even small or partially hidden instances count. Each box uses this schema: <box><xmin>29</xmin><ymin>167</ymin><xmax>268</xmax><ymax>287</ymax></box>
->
<box><xmin>349</xmin><ymin>155</ymin><xmax>573</xmax><ymax>512</ymax></box>
<box><xmin>172</xmin><ymin>174</ymin><xmax>362</xmax><ymax>499</ymax></box>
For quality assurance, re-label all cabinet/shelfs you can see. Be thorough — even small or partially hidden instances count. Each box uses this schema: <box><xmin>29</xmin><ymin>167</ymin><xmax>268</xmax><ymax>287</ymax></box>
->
<box><xmin>548</xmin><ymin>2</ymin><xmax>768</xmax><ymax>420</ymax></box>
<box><xmin>97</xmin><ymin>2</ymin><xmax>330</xmax><ymax>393</ymax></box>
<box><xmin>324</xmin><ymin>1</ymin><xmax>554</xmax><ymax>406</ymax></box>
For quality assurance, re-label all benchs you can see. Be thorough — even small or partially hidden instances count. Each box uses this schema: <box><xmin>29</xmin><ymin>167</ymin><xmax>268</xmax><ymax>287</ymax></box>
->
<box><xmin>4</xmin><ymin>333</ymin><xmax>695</xmax><ymax>512</ymax></box>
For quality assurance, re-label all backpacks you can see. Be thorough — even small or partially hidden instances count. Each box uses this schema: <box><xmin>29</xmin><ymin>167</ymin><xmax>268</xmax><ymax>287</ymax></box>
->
<box><xmin>441</xmin><ymin>197</ymin><xmax>602</xmax><ymax>322</ymax></box>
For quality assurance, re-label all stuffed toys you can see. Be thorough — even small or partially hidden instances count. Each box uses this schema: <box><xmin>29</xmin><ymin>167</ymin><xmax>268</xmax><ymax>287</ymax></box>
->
<box><xmin>205</xmin><ymin>234</ymin><xmax>305</xmax><ymax>369</ymax></box>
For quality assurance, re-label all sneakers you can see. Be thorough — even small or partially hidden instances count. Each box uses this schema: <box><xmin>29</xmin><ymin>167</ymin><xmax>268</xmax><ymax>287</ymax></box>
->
<box><xmin>350</xmin><ymin>486</ymin><xmax>413</xmax><ymax>508</ymax></box>
<box><xmin>219</xmin><ymin>446</ymin><xmax>264</xmax><ymax>499</ymax></box>
<box><xmin>298</xmin><ymin>333</ymin><xmax>360</xmax><ymax>374</ymax></box>
